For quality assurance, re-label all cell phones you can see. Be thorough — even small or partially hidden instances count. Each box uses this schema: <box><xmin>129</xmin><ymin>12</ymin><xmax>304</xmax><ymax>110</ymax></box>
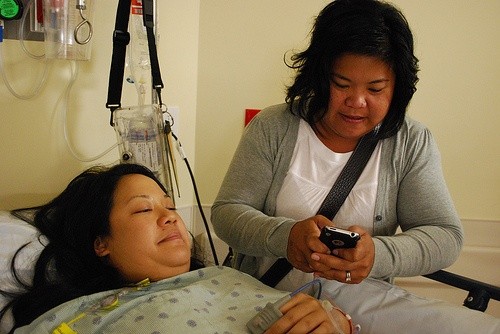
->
<box><xmin>320</xmin><ymin>226</ymin><xmax>360</xmax><ymax>252</ymax></box>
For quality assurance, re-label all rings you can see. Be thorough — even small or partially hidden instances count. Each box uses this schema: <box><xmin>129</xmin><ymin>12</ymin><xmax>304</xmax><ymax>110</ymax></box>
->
<box><xmin>346</xmin><ymin>270</ymin><xmax>352</xmax><ymax>285</ymax></box>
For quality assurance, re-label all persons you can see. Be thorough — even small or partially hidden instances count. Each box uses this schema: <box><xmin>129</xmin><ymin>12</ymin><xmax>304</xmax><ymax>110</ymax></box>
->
<box><xmin>6</xmin><ymin>164</ymin><xmax>360</xmax><ymax>333</ymax></box>
<box><xmin>210</xmin><ymin>0</ymin><xmax>465</xmax><ymax>297</ymax></box>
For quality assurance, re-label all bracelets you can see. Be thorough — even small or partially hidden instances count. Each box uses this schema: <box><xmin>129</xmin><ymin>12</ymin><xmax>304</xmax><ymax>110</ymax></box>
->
<box><xmin>335</xmin><ymin>305</ymin><xmax>355</xmax><ymax>332</ymax></box>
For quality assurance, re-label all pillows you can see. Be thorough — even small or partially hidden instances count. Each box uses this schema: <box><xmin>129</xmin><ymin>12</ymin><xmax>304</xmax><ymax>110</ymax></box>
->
<box><xmin>0</xmin><ymin>209</ymin><xmax>67</xmax><ymax>298</ymax></box>
